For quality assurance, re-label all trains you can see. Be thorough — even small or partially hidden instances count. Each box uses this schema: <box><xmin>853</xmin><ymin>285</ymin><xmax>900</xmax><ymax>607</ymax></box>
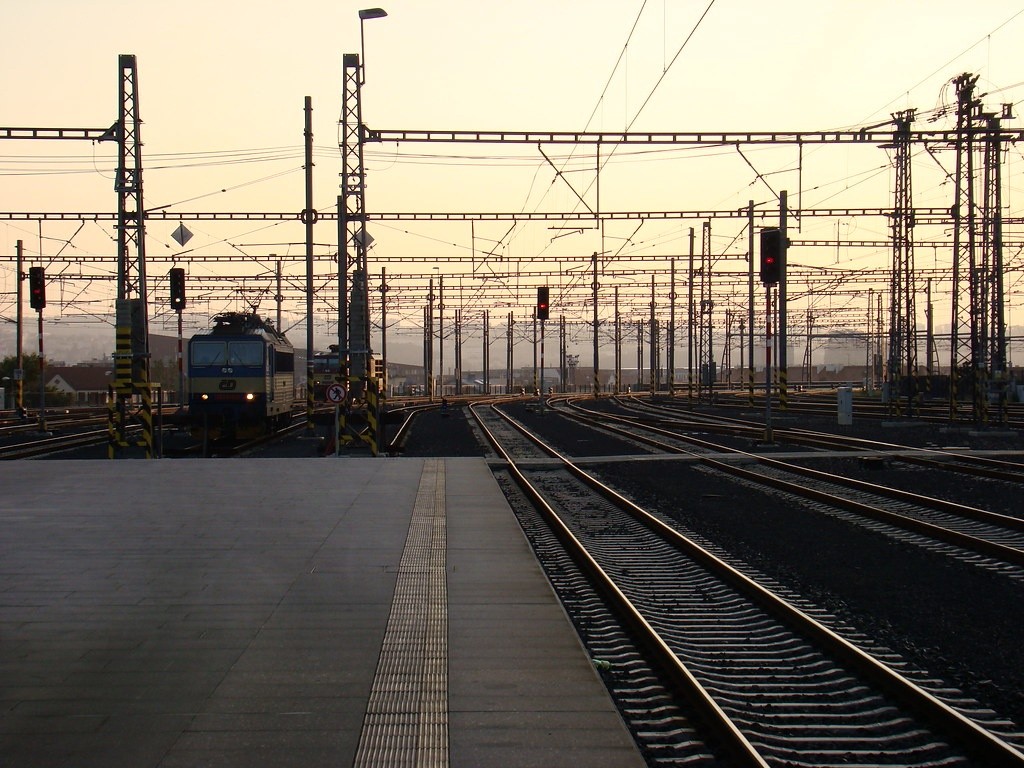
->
<box><xmin>313</xmin><ymin>342</ymin><xmax>388</xmax><ymax>402</ymax></box>
<box><xmin>187</xmin><ymin>303</ymin><xmax>297</xmax><ymax>435</ymax></box>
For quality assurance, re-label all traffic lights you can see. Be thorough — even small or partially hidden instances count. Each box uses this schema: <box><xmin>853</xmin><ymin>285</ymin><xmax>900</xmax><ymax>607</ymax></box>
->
<box><xmin>548</xmin><ymin>387</ymin><xmax>553</xmax><ymax>395</ymax></box>
<box><xmin>28</xmin><ymin>266</ymin><xmax>46</xmax><ymax>309</ymax></box>
<box><xmin>537</xmin><ymin>286</ymin><xmax>550</xmax><ymax>319</ymax></box>
<box><xmin>521</xmin><ymin>387</ymin><xmax>525</xmax><ymax>395</ymax></box>
<box><xmin>169</xmin><ymin>267</ymin><xmax>186</xmax><ymax>310</ymax></box>
<box><xmin>760</xmin><ymin>227</ymin><xmax>781</xmax><ymax>284</ymax></box>
